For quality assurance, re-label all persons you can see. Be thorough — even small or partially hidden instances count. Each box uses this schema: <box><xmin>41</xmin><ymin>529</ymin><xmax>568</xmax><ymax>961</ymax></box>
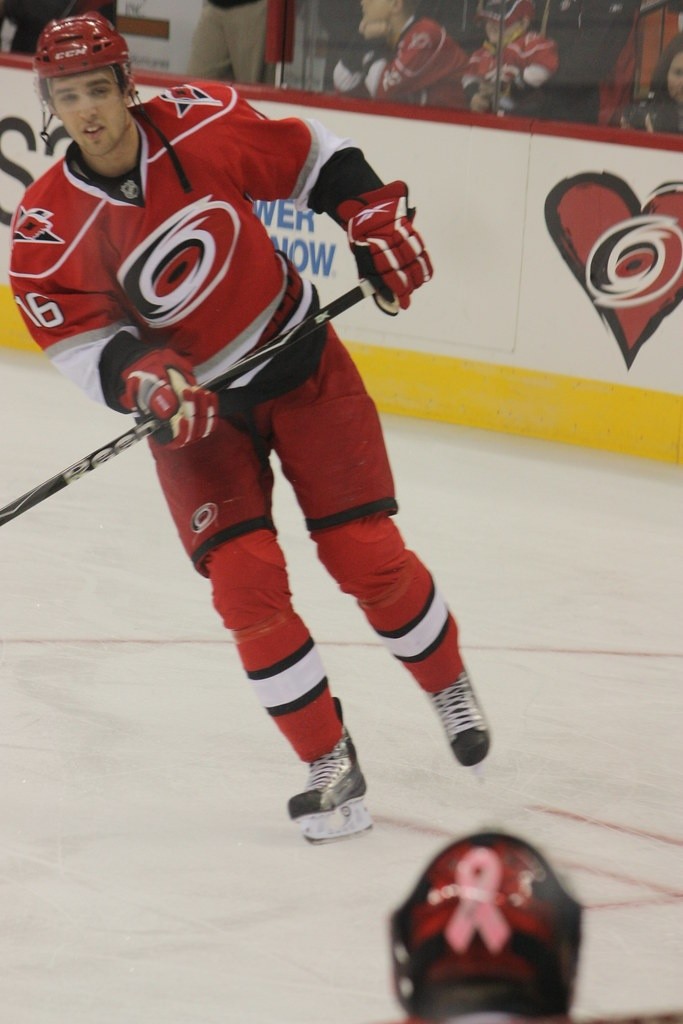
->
<box><xmin>619</xmin><ymin>30</ymin><xmax>682</xmax><ymax>134</ymax></box>
<box><xmin>378</xmin><ymin>824</ymin><xmax>587</xmax><ymax>1024</ymax></box>
<box><xmin>335</xmin><ymin>4</ymin><xmax>459</xmax><ymax>105</ymax></box>
<box><xmin>10</xmin><ymin>14</ymin><xmax>494</xmax><ymax>842</ymax></box>
<box><xmin>442</xmin><ymin>0</ymin><xmax>553</xmax><ymax>115</ymax></box>
<box><xmin>183</xmin><ymin>0</ymin><xmax>268</xmax><ymax>86</ymax></box>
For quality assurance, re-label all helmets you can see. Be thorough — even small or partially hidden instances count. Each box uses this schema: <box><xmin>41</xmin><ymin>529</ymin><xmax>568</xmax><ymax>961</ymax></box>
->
<box><xmin>33</xmin><ymin>10</ymin><xmax>130</xmax><ymax>97</ymax></box>
<box><xmin>390</xmin><ymin>832</ymin><xmax>581</xmax><ymax>1018</ymax></box>
<box><xmin>476</xmin><ymin>0</ymin><xmax>534</xmax><ymax>30</ymax></box>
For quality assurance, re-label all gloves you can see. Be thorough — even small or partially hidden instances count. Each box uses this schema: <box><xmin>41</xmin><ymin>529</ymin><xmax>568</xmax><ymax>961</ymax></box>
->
<box><xmin>117</xmin><ymin>347</ymin><xmax>217</xmax><ymax>449</ymax></box>
<box><xmin>337</xmin><ymin>179</ymin><xmax>434</xmax><ymax>316</ymax></box>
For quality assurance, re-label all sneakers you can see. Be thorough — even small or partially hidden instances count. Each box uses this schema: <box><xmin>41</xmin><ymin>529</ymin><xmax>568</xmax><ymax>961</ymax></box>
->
<box><xmin>428</xmin><ymin>668</ymin><xmax>491</xmax><ymax>779</ymax></box>
<box><xmin>287</xmin><ymin>697</ymin><xmax>374</xmax><ymax>845</ymax></box>
